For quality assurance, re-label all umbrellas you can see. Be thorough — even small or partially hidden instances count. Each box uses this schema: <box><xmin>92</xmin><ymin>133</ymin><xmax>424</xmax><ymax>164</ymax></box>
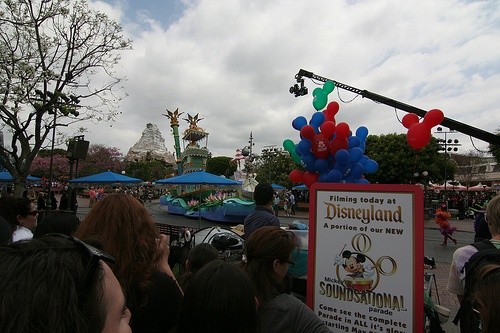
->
<box><xmin>156</xmin><ymin>172</ymin><xmax>241</xmax><ymax>229</ymax></box>
<box><xmin>68</xmin><ymin>172</ymin><xmax>143</xmax><ymax>182</ymax></box>
<box><xmin>415</xmin><ymin>180</ymin><xmax>492</xmax><ymax>192</ymax></box>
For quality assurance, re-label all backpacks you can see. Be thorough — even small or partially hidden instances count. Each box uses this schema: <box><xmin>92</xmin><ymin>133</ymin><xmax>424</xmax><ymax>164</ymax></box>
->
<box><xmin>461</xmin><ymin>239</ymin><xmax>500</xmax><ymax>333</ymax></box>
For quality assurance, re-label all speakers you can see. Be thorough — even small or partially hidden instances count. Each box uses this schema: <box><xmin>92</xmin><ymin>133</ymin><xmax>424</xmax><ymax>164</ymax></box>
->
<box><xmin>74</xmin><ymin>141</ymin><xmax>89</xmax><ymax>159</ymax></box>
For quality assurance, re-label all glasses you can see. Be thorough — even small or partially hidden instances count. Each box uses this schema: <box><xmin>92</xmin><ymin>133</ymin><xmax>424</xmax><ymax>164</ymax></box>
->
<box><xmin>26</xmin><ymin>210</ymin><xmax>39</xmax><ymax>216</ymax></box>
<box><xmin>285</xmin><ymin>260</ymin><xmax>295</xmax><ymax>269</ymax></box>
<box><xmin>484</xmin><ymin>212</ymin><xmax>489</xmax><ymax>223</ymax></box>
<box><xmin>42</xmin><ymin>232</ymin><xmax>117</xmax><ymax>303</ymax></box>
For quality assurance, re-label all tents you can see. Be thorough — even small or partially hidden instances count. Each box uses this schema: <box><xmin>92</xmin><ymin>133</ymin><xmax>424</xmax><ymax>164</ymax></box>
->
<box><xmin>0</xmin><ymin>172</ymin><xmax>50</xmax><ymax>181</ymax></box>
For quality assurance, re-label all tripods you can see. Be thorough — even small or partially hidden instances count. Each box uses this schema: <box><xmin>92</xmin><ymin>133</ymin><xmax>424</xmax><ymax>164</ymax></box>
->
<box><xmin>425</xmin><ymin>268</ymin><xmax>440</xmax><ymax>321</ymax></box>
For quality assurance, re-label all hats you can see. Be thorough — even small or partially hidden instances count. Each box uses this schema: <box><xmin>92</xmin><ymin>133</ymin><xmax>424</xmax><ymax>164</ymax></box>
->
<box><xmin>254</xmin><ymin>183</ymin><xmax>274</xmax><ymax>205</ymax></box>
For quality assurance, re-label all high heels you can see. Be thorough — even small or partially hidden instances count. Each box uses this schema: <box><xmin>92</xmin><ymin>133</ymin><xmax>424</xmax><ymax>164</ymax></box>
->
<box><xmin>453</xmin><ymin>239</ymin><xmax>458</xmax><ymax>245</ymax></box>
<box><xmin>440</xmin><ymin>242</ymin><xmax>447</xmax><ymax>248</ymax></box>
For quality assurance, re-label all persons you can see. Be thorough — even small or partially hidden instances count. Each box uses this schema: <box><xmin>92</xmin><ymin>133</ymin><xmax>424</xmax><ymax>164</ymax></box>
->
<box><xmin>447</xmin><ymin>195</ymin><xmax>500</xmax><ymax>333</ymax></box>
<box><xmin>424</xmin><ymin>190</ymin><xmax>500</xmax><ymax>219</ymax></box>
<box><xmin>434</xmin><ymin>204</ymin><xmax>458</xmax><ymax>246</ymax></box>
<box><xmin>0</xmin><ymin>183</ymin><xmax>332</xmax><ymax>333</ymax></box>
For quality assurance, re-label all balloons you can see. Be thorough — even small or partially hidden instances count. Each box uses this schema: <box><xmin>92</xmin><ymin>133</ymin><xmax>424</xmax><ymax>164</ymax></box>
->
<box><xmin>283</xmin><ymin>80</ymin><xmax>378</xmax><ymax>190</ymax></box>
<box><xmin>402</xmin><ymin>110</ymin><xmax>444</xmax><ymax>150</ymax></box>
